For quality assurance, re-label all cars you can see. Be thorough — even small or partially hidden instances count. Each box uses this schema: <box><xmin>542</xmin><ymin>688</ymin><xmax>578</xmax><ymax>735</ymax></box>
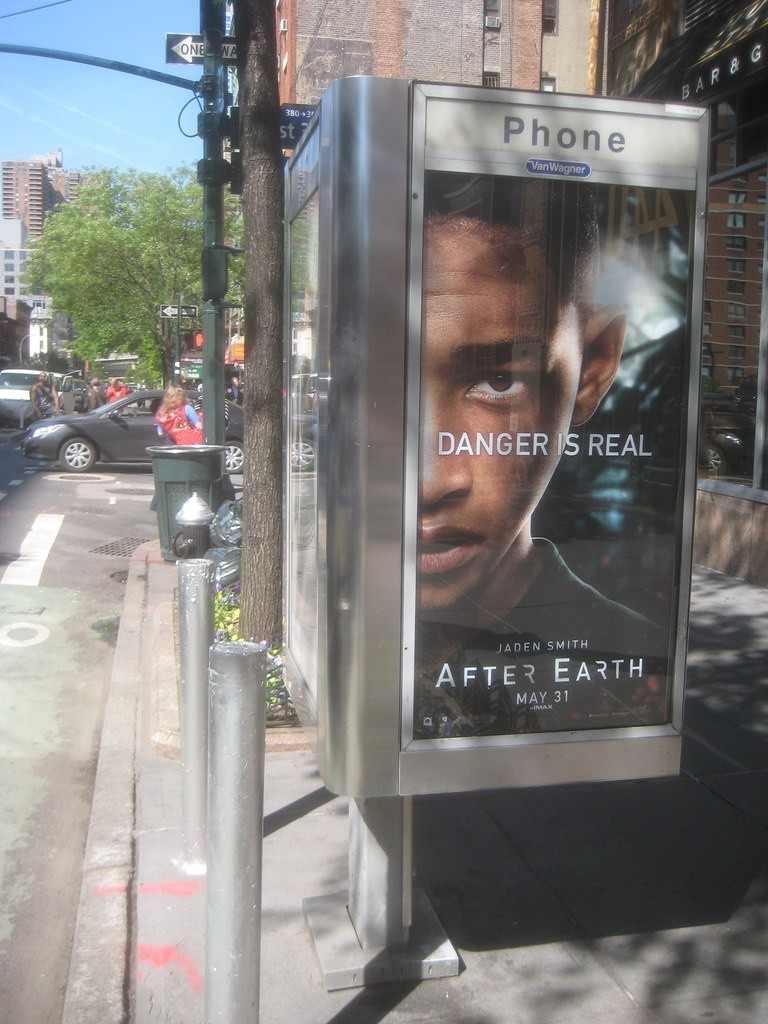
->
<box><xmin>18</xmin><ymin>389</ymin><xmax>245</xmax><ymax>475</ymax></box>
<box><xmin>697</xmin><ymin>389</ymin><xmax>755</xmax><ymax>480</ymax></box>
<box><xmin>281</xmin><ymin>372</ymin><xmax>331</xmax><ymax>475</ymax></box>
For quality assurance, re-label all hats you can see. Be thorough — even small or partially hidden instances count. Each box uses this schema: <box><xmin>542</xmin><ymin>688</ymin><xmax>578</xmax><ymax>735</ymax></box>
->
<box><xmin>117</xmin><ymin>382</ymin><xmax>126</xmax><ymax>387</ymax></box>
<box><xmin>92</xmin><ymin>377</ymin><xmax>101</xmax><ymax>386</ymax></box>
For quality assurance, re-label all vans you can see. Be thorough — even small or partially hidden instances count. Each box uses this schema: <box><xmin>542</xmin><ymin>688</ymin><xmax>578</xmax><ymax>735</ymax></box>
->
<box><xmin>0</xmin><ymin>369</ymin><xmax>74</xmax><ymax>426</ymax></box>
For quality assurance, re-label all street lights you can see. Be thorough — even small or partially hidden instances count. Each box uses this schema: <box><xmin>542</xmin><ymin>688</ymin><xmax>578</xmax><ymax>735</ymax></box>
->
<box><xmin>18</xmin><ymin>332</ymin><xmax>35</xmax><ymax>369</ymax></box>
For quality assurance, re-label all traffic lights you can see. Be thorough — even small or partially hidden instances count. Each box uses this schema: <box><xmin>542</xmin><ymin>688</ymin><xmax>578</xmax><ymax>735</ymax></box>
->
<box><xmin>192</xmin><ymin>330</ymin><xmax>203</xmax><ymax>351</ymax></box>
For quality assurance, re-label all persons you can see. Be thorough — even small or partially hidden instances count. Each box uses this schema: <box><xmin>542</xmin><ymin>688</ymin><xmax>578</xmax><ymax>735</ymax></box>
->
<box><xmin>29</xmin><ymin>372</ymin><xmax>147</xmax><ymax>422</ymax></box>
<box><xmin>150</xmin><ymin>377</ymin><xmax>243</xmax><ymax>511</ymax></box>
<box><xmin>413</xmin><ymin>169</ymin><xmax>671</xmax><ymax>741</ymax></box>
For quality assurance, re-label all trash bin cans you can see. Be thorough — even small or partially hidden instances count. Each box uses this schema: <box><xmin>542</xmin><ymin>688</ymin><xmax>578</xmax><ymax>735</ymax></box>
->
<box><xmin>145</xmin><ymin>444</ymin><xmax>228</xmax><ymax>562</ymax></box>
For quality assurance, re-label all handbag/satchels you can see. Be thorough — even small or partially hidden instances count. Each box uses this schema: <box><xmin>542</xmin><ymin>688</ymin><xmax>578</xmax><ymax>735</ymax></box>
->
<box><xmin>38</xmin><ymin>402</ymin><xmax>57</xmax><ymax>415</ymax></box>
<box><xmin>172</xmin><ymin>406</ymin><xmax>203</xmax><ymax>445</ymax></box>
